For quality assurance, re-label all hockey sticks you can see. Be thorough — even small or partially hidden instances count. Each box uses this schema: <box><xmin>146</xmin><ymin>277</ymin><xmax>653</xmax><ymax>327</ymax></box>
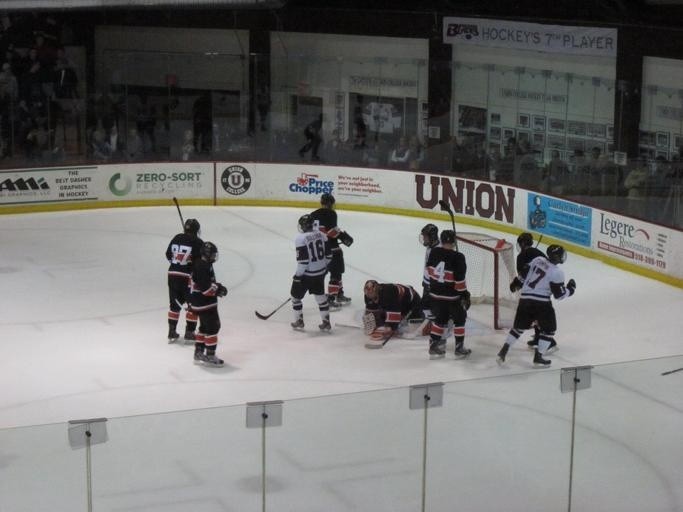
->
<box><xmin>255</xmin><ymin>298</ymin><xmax>291</xmax><ymax>320</ymax></box>
<box><xmin>365</xmin><ymin>297</ymin><xmax>423</xmax><ymax>349</ymax></box>
<box><xmin>438</xmin><ymin>200</ymin><xmax>458</xmax><ymax>252</ymax></box>
<box><xmin>336</xmin><ymin>320</ymin><xmax>428</xmax><ymax>339</ymax></box>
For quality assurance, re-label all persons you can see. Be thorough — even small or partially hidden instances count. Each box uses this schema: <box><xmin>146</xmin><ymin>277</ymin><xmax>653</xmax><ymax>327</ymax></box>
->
<box><xmin>290</xmin><ymin>214</ymin><xmax>333</xmax><ymax>330</ymax></box>
<box><xmin>310</xmin><ymin>193</ymin><xmax>353</xmax><ymax>307</ymax></box>
<box><xmin>364</xmin><ymin>223</ymin><xmax>472</xmax><ymax>356</ymax></box>
<box><xmin>165</xmin><ymin>219</ymin><xmax>205</xmax><ymax>340</ymax></box>
<box><xmin>497</xmin><ymin>245</ymin><xmax>576</xmax><ymax>364</ymax></box>
<box><xmin>190</xmin><ymin>242</ymin><xmax>227</xmax><ymax>364</ymax></box>
<box><xmin>510</xmin><ymin>233</ymin><xmax>557</xmax><ymax>349</ymax></box>
<box><xmin>1</xmin><ymin>10</ymin><xmax>683</xmax><ymax>231</ymax></box>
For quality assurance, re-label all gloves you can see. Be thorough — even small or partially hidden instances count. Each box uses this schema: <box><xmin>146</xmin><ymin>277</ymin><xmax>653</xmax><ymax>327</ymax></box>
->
<box><xmin>339</xmin><ymin>232</ymin><xmax>353</xmax><ymax>246</ymax></box>
<box><xmin>567</xmin><ymin>278</ymin><xmax>576</xmax><ymax>297</ymax></box>
<box><xmin>509</xmin><ymin>277</ymin><xmax>522</xmax><ymax>292</ymax></box>
<box><xmin>218</xmin><ymin>283</ymin><xmax>229</xmax><ymax>298</ymax></box>
<box><xmin>460</xmin><ymin>290</ymin><xmax>471</xmax><ymax>310</ymax></box>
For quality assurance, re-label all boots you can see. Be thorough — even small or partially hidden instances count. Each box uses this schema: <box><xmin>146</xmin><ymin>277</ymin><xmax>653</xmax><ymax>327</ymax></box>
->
<box><xmin>193</xmin><ymin>347</ymin><xmax>225</xmax><ymax>365</ymax></box>
<box><xmin>526</xmin><ymin>333</ymin><xmax>557</xmax><ymax>349</ymax></box>
<box><xmin>317</xmin><ymin>316</ymin><xmax>332</xmax><ymax>329</ymax></box>
<box><xmin>291</xmin><ymin>316</ymin><xmax>303</xmax><ymax>328</ymax></box>
<box><xmin>428</xmin><ymin>338</ymin><xmax>445</xmax><ymax>354</ymax></box>
<box><xmin>325</xmin><ymin>289</ymin><xmax>351</xmax><ymax>306</ymax></box>
<box><xmin>498</xmin><ymin>342</ymin><xmax>510</xmax><ymax>362</ymax></box>
<box><xmin>454</xmin><ymin>341</ymin><xmax>472</xmax><ymax>355</ymax></box>
<box><xmin>167</xmin><ymin>325</ymin><xmax>180</xmax><ymax>339</ymax></box>
<box><xmin>533</xmin><ymin>350</ymin><xmax>552</xmax><ymax>364</ymax></box>
<box><xmin>183</xmin><ymin>330</ymin><xmax>196</xmax><ymax>341</ymax></box>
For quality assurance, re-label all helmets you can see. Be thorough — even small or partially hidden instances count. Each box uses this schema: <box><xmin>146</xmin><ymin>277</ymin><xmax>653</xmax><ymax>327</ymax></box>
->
<box><xmin>441</xmin><ymin>229</ymin><xmax>457</xmax><ymax>246</ymax></box>
<box><xmin>199</xmin><ymin>241</ymin><xmax>218</xmax><ymax>264</ymax></box>
<box><xmin>364</xmin><ymin>281</ymin><xmax>381</xmax><ymax>304</ymax></box>
<box><xmin>296</xmin><ymin>215</ymin><xmax>315</xmax><ymax>232</ymax></box>
<box><xmin>321</xmin><ymin>191</ymin><xmax>335</xmax><ymax>208</ymax></box>
<box><xmin>183</xmin><ymin>218</ymin><xmax>200</xmax><ymax>236</ymax></box>
<box><xmin>418</xmin><ymin>223</ymin><xmax>439</xmax><ymax>247</ymax></box>
<box><xmin>515</xmin><ymin>231</ymin><xmax>534</xmax><ymax>249</ymax></box>
<box><xmin>545</xmin><ymin>243</ymin><xmax>567</xmax><ymax>265</ymax></box>
